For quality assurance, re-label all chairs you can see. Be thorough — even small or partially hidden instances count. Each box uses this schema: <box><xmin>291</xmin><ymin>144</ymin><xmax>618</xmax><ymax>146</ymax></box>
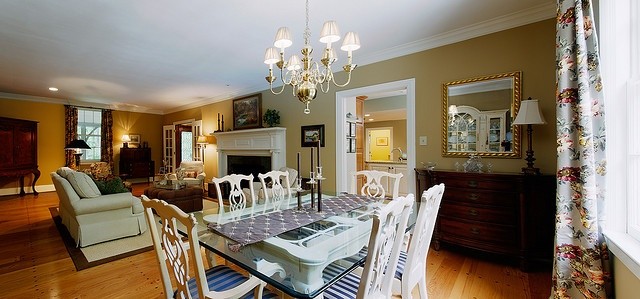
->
<box><xmin>138</xmin><ymin>194</ymin><xmax>287</xmax><ymax>299</ymax></box>
<box><xmin>361</xmin><ymin>182</ymin><xmax>446</xmax><ymax>299</ymax></box>
<box><xmin>258</xmin><ymin>170</ymin><xmax>331</xmax><ymax>240</ymax></box>
<box><xmin>212</xmin><ymin>174</ymin><xmax>256</xmax><ymax>213</ymax></box>
<box><xmin>335</xmin><ymin>170</ymin><xmax>403</xmax><ymax>267</ymax></box>
<box><xmin>275</xmin><ymin>194</ymin><xmax>413</xmax><ymax>299</ymax></box>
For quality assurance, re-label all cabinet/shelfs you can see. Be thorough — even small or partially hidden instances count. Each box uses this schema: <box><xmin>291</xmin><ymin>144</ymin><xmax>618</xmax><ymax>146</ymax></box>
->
<box><xmin>119</xmin><ymin>148</ymin><xmax>155</xmax><ymax>182</ymax></box>
<box><xmin>480</xmin><ymin>109</ymin><xmax>509</xmax><ymax>153</ymax></box>
<box><xmin>0</xmin><ymin>116</ymin><xmax>40</xmax><ymax>198</ymax></box>
<box><xmin>413</xmin><ymin>168</ymin><xmax>556</xmax><ymax>274</ymax></box>
<box><xmin>445</xmin><ymin>106</ymin><xmax>480</xmax><ymax>152</ymax></box>
<box><xmin>210</xmin><ymin>127</ymin><xmax>287</xmax><ymax>178</ymax></box>
<box><xmin>366</xmin><ymin>161</ymin><xmax>407</xmax><ymax>199</ymax></box>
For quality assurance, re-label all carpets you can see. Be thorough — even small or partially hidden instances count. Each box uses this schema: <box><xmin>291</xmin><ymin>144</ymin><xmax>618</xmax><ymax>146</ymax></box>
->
<box><xmin>48</xmin><ymin>195</ymin><xmax>229</xmax><ymax>273</ymax></box>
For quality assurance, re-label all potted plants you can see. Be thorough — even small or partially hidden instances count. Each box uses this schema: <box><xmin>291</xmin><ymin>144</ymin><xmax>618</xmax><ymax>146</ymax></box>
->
<box><xmin>263</xmin><ymin>109</ymin><xmax>280</xmax><ymax>127</ymax></box>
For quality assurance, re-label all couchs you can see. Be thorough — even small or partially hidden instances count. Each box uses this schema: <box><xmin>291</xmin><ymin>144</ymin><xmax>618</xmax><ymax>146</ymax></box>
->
<box><xmin>173</xmin><ymin>162</ymin><xmax>206</xmax><ymax>194</ymax></box>
<box><xmin>144</xmin><ymin>185</ymin><xmax>203</xmax><ymax>217</ymax></box>
<box><xmin>207</xmin><ymin>182</ymin><xmax>230</xmax><ymax>198</ymax></box>
<box><xmin>243</xmin><ymin>166</ymin><xmax>299</xmax><ymax>208</ymax></box>
<box><xmin>49</xmin><ymin>167</ymin><xmax>146</xmax><ymax>248</ymax></box>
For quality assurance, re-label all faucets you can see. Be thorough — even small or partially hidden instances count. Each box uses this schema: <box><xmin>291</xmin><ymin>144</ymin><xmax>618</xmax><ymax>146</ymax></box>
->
<box><xmin>390</xmin><ymin>148</ymin><xmax>402</xmax><ymax>162</ymax></box>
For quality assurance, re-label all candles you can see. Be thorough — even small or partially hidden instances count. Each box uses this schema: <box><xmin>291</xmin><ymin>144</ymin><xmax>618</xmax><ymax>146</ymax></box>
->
<box><xmin>315</xmin><ymin>140</ymin><xmax>326</xmax><ymax>213</ymax></box>
<box><xmin>297</xmin><ymin>152</ymin><xmax>301</xmax><ymax>210</ymax></box>
<box><xmin>215</xmin><ymin>113</ymin><xmax>221</xmax><ymax>133</ymax></box>
<box><xmin>307</xmin><ymin>148</ymin><xmax>317</xmax><ymax>209</ymax></box>
<box><xmin>221</xmin><ymin>115</ymin><xmax>224</xmax><ymax>131</ymax></box>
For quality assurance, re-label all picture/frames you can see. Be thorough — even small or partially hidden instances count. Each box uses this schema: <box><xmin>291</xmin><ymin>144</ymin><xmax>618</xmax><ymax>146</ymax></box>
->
<box><xmin>129</xmin><ymin>133</ymin><xmax>142</xmax><ymax>145</ymax></box>
<box><xmin>300</xmin><ymin>124</ymin><xmax>325</xmax><ymax>148</ymax></box>
<box><xmin>376</xmin><ymin>136</ymin><xmax>388</xmax><ymax>146</ymax></box>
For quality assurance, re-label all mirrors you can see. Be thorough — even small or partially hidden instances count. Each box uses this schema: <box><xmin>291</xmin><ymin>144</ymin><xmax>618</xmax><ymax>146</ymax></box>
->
<box><xmin>440</xmin><ymin>69</ymin><xmax>522</xmax><ymax>160</ymax></box>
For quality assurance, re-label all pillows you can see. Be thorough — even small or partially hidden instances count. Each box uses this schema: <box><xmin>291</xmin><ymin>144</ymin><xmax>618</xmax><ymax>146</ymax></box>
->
<box><xmin>94</xmin><ymin>176</ymin><xmax>129</xmax><ymax>195</ymax></box>
<box><xmin>261</xmin><ymin>179</ymin><xmax>282</xmax><ymax>189</ymax></box>
<box><xmin>183</xmin><ymin>171</ymin><xmax>196</xmax><ymax>178</ymax></box>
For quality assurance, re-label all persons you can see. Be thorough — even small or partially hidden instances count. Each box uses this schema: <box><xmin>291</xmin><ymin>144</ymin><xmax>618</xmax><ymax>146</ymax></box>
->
<box><xmin>505</xmin><ymin>128</ymin><xmax>512</xmax><ymax>152</ymax></box>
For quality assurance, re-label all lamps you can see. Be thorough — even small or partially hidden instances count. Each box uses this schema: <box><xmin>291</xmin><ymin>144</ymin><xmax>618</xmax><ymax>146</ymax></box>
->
<box><xmin>511</xmin><ymin>97</ymin><xmax>548</xmax><ymax>175</ymax></box>
<box><xmin>64</xmin><ymin>139</ymin><xmax>91</xmax><ymax>170</ymax></box>
<box><xmin>264</xmin><ymin>0</ymin><xmax>362</xmax><ymax>114</ymax></box>
<box><xmin>197</xmin><ymin>135</ymin><xmax>208</xmax><ymax>191</ymax></box>
<box><xmin>447</xmin><ymin>106</ymin><xmax>461</xmax><ymax>127</ymax></box>
